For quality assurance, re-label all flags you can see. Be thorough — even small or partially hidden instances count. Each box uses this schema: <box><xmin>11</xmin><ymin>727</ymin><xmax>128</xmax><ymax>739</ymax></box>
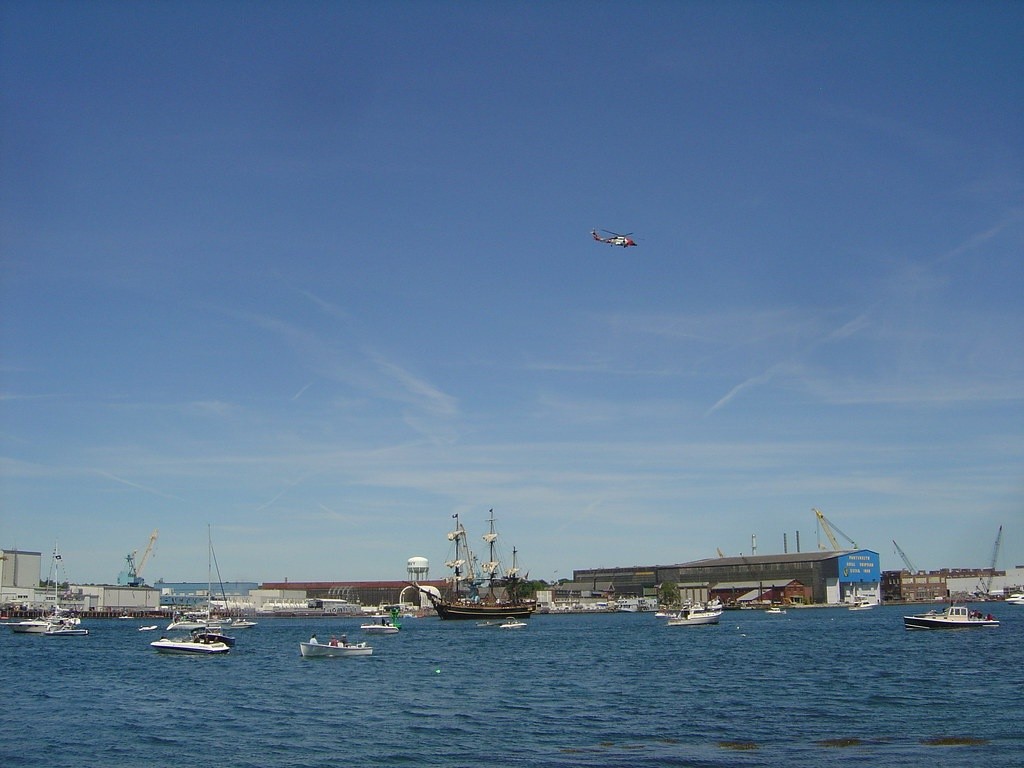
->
<box><xmin>55</xmin><ymin>555</ymin><xmax>61</xmax><ymax>560</ymax></box>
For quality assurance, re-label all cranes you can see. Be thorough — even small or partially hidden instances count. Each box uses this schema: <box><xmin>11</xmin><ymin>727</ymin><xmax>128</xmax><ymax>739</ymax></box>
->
<box><xmin>125</xmin><ymin>527</ymin><xmax>160</xmax><ymax>587</ymax></box>
<box><xmin>892</xmin><ymin>540</ymin><xmax>918</xmax><ymax>575</ymax></box>
<box><xmin>976</xmin><ymin>524</ymin><xmax>1003</xmax><ymax>598</ymax></box>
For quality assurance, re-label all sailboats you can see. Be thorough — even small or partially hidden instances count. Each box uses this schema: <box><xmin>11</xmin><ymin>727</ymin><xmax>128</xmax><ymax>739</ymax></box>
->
<box><xmin>0</xmin><ymin>541</ymin><xmax>89</xmax><ymax>635</ymax></box>
<box><xmin>166</xmin><ymin>524</ymin><xmax>258</xmax><ymax>630</ymax></box>
<box><xmin>402</xmin><ymin>508</ymin><xmax>541</xmax><ymax>620</ymax></box>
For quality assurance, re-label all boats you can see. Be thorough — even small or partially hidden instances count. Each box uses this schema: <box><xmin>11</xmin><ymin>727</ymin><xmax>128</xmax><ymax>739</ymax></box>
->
<box><xmin>500</xmin><ymin>616</ymin><xmax>527</xmax><ymax>629</ymax></box>
<box><xmin>138</xmin><ymin>625</ymin><xmax>158</xmax><ymax>632</ymax></box>
<box><xmin>902</xmin><ymin>606</ymin><xmax>1001</xmax><ymax>630</ymax></box>
<box><xmin>765</xmin><ymin>608</ymin><xmax>787</xmax><ymax>614</ymax></box>
<box><xmin>655</xmin><ymin>598</ymin><xmax>723</xmax><ymax>626</ymax></box>
<box><xmin>195</xmin><ymin>633</ymin><xmax>236</xmax><ymax>646</ymax></box>
<box><xmin>119</xmin><ymin>611</ymin><xmax>135</xmax><ymax>618</ymax></box>
<box><xmin>360</xmin><ymin>623</ymin><xmax>399</xmax><ymax>634</ymax></box>
<box><xmin>300</xmin><ymin>642</ymin><xmax>373</xmax><ymax>657</ymax></box>
<box><xmin>150</xmin><ymin>638</ymin><xmax>230</xmax><ymax>655</ymax></box>
<box><xmin>1005</xmin><ymin>594</ymin><xmax>1024</xmax><ymax>605</ymax></box>
<box><xmin>476</xmin><ymin>621</ymin><xmax>502</xmax><ymax>626</ymax></box>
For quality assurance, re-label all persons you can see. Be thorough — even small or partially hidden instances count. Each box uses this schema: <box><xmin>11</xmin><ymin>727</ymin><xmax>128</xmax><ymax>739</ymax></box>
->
<box><xmin>310</xmin><ymin>634</ymin><xmax>318</xmax><ymax>644</ymax></box>
<box><xmin>970</xmin><ymin>610</ymin><xmax>994</xmax><ymax>621</ymax></box>
<box><xmin>193</xmin><ymin>634</ymin><xmax>218</xmax><ymax>645</ymax></box>
<box><xmin>329</xmin><ymin>634</ymin><xmax>349</xmax><ymax>648</ymax></box>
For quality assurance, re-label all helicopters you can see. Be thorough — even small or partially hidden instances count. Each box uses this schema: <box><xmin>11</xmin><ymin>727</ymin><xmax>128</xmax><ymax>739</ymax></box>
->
<box><xmin>590</xmin><ymin>227</ymin><xmax>637</xmax><ymax>248</ymax></box>
